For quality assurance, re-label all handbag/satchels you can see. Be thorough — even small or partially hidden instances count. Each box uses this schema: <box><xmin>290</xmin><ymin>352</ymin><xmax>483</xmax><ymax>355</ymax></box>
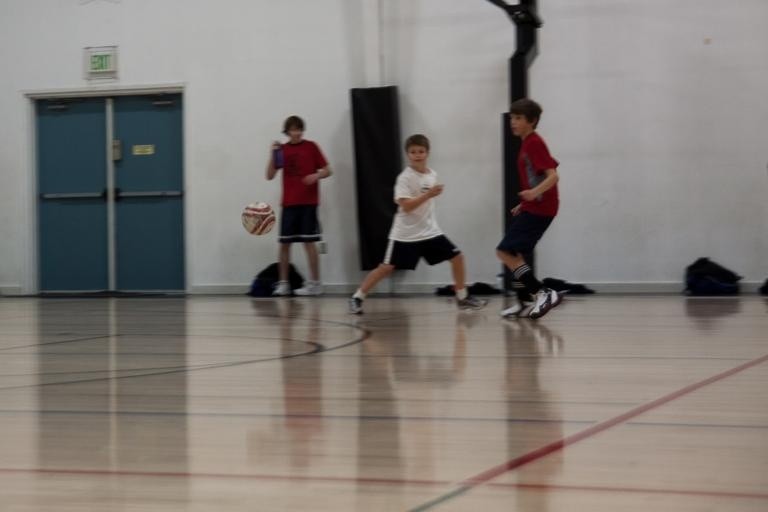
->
<box><xmin>685</xmin><ymin>257</ymin><xmax>743</xmax><ymax>298</ymax></box>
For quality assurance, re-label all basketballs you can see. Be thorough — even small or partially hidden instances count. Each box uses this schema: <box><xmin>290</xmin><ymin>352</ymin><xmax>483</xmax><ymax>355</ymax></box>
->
<box><xmin>241</xmin><ymin>202</ymin><xmax>275</xmax><ymax>235</ymax></box>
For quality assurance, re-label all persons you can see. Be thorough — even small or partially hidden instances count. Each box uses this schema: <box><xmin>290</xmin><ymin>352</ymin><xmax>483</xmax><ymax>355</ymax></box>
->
<box><xmin>265</xmin><ymin>116</ymin><xmax>333</xmax><ymax>297</ymax></box>
<box><xmin>347</xmin><ymin>134</ymin><xmax>488</xmax><ymax>314</ymax></box>
<box><xmin>495</xmin><ymin>98</ymin><xmax>562</xmax><ymax>319</ymax></box>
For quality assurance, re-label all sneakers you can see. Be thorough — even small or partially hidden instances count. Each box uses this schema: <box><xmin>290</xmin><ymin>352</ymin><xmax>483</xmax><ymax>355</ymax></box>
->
<box><xmin>272</xmin><ymin>283</ymin><xmax>292</xmax><ymax>295</ymax></box>
<box><xmin>456</xmin><ymin>294</ymin><xmax>488</xmax><ymax>312</ymax></box>
<box><xmin>294</xmin><ymin>281</ymin><xmax>326</xmax><ymax>297</ymax></box>
<box><xmin>346</xmin><ymin>298</ymin><xmax>366</xmax><ymax>315</ymax></box>
<box><xmin>502</xmin><ymin>283</ymin><xmax>560</xmax><ymax>320</ymax></box>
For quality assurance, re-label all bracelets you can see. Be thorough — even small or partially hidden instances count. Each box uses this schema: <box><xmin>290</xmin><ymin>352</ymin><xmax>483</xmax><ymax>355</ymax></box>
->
<box><xmin>317</xmin><ymin>167</ymin><xmax>326</xmax><ymax>179</ymax></box>
